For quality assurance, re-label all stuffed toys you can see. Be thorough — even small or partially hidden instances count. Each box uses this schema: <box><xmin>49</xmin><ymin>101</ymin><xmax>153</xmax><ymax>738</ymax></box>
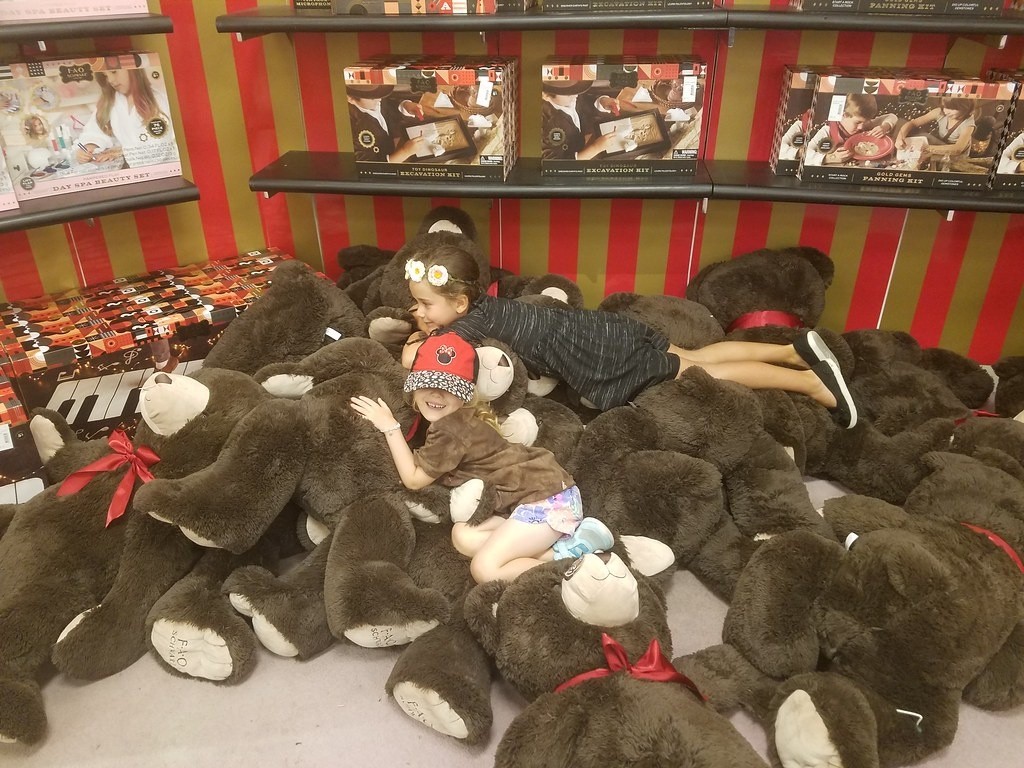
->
<box><xmin>0</xmin><ymin>208</ymin><xmax>1024</xmax><ymax>768</ymax></box>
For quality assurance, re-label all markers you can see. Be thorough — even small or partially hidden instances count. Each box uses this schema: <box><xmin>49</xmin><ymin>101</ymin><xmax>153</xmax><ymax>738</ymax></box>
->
<box><xmin>73</xmin><ymin>138</ymin><xmax>97</xmax><ymax>163</ymax></box>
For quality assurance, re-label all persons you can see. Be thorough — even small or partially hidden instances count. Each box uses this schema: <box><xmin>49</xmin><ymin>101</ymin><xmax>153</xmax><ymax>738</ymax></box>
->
<box><xmin>542</xmin><ymin>76</ymin><xmax>623</xmax><ymax>166</ymax></box>
<box><xmin>23</xmin><ymin>66</ymin><xmax>180</xmax><ymax>172</ymax></box>
<box><xmin>347</xmin><ymin>332</ymin><xmax>613</xmax><ymax>583</ymax></box>
<box><xmin>784</xmin><ymin>89</ymin><xmax>1024</xmax><ymax>181</ymax></box>
<box><xmin>404</xmin><ymin>244</ymin><xmax>860</xmax><ymax>430</ymax></box>
<box><xmin>346</xmin><ymin>84</ymin><xmax>431</xmax><ymax>165</ymax></box>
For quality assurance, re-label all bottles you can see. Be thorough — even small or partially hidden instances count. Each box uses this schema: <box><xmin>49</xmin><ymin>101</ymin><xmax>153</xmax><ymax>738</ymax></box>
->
<box><xmin>939</xmin><ymin>154</ymin><xmax>951</xmax><ymax>173</ymax></box>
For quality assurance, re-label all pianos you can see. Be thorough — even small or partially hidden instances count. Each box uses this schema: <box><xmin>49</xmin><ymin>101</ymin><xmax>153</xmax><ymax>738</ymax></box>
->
<box><xmin>43</xmin><ymin>357</ymin><xmax>205</xmax><ymax>426</ymax></box>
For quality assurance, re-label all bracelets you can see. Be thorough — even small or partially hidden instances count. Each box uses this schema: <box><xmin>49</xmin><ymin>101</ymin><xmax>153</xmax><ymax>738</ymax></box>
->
<box><xmin>380</xmin><ymin>423</ymin><xmax>401</xmax><ymax>432</ymax></box>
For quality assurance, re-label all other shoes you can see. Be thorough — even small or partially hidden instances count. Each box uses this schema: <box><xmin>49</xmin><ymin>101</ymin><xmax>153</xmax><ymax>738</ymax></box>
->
<box><xmin>792</xmin><ymin>330</ymin><xmax>841</xmax><ymax>376</ymax></box>
<box><xmin>811</xmin><ymin>358</ymin><xmax>858</xmax><ymax>429</ymax></box>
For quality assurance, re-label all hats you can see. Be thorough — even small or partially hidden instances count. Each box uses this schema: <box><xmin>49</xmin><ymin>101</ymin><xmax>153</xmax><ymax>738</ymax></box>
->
<box><xmin>403</xmin><ymin>331</ymin><xmax>480</xmax><ymax>404</ymax></box>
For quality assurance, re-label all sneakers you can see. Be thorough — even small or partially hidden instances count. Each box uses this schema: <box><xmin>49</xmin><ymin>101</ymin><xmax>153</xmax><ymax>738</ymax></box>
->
<box><xmin>552</xmin><ymin>516</ymin><xmax>615</xmax><ymax>561</ymax></box>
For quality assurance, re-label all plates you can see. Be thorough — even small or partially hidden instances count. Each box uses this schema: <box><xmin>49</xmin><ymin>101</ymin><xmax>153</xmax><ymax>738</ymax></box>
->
<box><xmin>845</xmin><ymin>133</ymin><xmax>894</xmax><ymax>160</ymax></box>
